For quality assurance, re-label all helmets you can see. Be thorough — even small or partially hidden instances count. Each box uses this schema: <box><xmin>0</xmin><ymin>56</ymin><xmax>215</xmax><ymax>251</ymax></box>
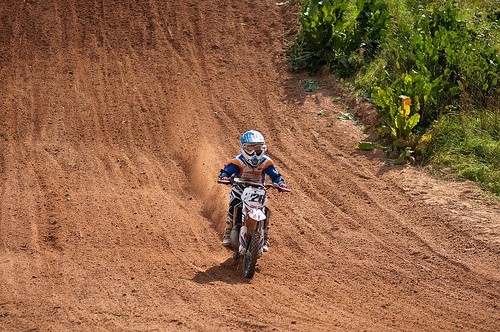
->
<box><xmin>240</xmin><ymin>130</ymin><xmax>266</xmax><ymax>166</ymax></box>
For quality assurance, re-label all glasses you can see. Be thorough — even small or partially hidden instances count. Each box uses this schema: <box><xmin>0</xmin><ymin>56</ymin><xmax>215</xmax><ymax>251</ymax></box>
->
<box><xmin>244</xmin><ymin>145</ymin><xmax>262</xmax><ymax>151</ymax></box>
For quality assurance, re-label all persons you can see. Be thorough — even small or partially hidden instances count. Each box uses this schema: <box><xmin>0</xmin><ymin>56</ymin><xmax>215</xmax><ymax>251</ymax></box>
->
<box><xmin>217</xmin><ymin>130</ymin><xmax>287</xmax><ymax>252</ymax></box>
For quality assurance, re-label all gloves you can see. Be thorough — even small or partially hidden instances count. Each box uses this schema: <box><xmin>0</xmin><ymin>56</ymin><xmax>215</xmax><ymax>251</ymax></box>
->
<box><xmin>278</xmin><ymin>183</ymin><xmax>287</xmax><ymax>193</ymax></box>
<box><xmin>221</xmin><ymin>177</ymin><xmax>230</xmax><ymax>185</ymax></box>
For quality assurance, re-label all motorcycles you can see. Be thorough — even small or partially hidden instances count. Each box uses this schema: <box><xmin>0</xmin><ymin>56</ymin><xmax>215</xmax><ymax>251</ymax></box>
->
<box><xmin>218</xmin><ymin>177</ymin><xmax>291</xmax><ymax>280</ymax></box>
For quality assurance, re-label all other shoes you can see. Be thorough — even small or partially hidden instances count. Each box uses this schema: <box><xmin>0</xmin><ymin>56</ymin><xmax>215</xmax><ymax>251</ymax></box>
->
<box><xmin>263</xmin><ymin>240</ymin><xmax>269</xmax><ymax>251</ymax></box>
<box><xmin>222</xmin><ymin>232</ymin><xmax>231</xmax><ymax>246</ymax></box>
